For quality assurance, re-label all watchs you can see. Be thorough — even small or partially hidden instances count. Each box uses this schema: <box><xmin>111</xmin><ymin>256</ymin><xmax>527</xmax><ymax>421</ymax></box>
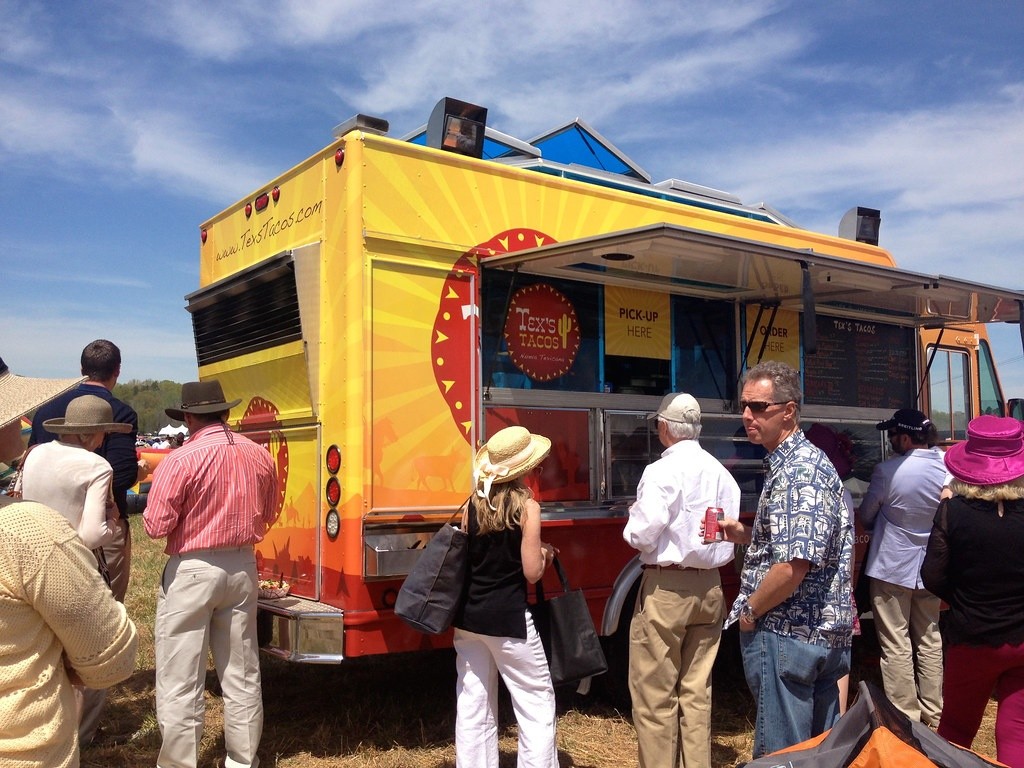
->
<box><xmin>742</xmin><ymin>603</ymin><xmax>753</xmax><ymax>618</ymax></box>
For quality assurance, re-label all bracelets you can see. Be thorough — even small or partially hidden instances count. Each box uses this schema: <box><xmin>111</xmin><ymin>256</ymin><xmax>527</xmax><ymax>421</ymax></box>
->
<box><xmin>741</xmin><ymin>611</ymin><xmax>755</xmax><ymax>624</ymax></box>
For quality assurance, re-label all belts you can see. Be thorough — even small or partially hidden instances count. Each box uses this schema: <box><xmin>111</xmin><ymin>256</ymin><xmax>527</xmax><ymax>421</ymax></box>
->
<box><xmin>644</xmin><ymin>564</ymin><xmax>706</xmax><ymax>571</ymax></box>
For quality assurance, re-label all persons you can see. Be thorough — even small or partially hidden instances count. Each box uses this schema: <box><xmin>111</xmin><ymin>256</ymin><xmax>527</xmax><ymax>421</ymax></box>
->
<box><xmin>621</xmin><ymin>392</ymin><xmax>741</xmax><ymax>768</ymax></box>
<box><xmin>26</xmin><ymin>339</ymin><xmax>141</xmax><ymax>604</ymax></box>
<box><xmin>0</xmin><ymin>358</ymin><xmax>140</xmax><ymax>768</ymax></box>
<box><xmin>135</xmin><ymin>432</ymin><xmax>184</xmax><ymax>449</ymax></box>
<box><xmin>142</xmin><ymin>378</ymin><xmax>276</xmax><ymax>768</ymax></box>
<box><xmin>441</xmin><ymin>425</ymin><xmax>562</xmax><ymax>768</ymax></box>
<box><xmin>919</xmin><ymin>415</ymin><xmax>1024</xmax><ymax>767</ymax></box>
<box><xmin>699</xmin><ymin>359</ymin><xmax>853</xmax><ymax>761</ymax></box>
<box><xmin>858</xmin><ymin>408</ymin><xmax>955</xmax><ymax>734</ymax></box>
<box><xmin>14</xmin><ymin>394</ymin><xmax>134</xmax><ymax>749</ymax></box>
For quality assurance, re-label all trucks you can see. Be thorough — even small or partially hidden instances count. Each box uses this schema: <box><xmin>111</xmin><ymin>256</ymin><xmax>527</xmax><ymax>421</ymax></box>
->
<box><xmin>179</xmin><ymin>94</ymin><xmax>1024</xmax><ymax>717</ymax></box>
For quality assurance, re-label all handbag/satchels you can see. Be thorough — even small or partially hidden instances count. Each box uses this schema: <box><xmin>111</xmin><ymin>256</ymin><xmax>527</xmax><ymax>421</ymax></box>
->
<box><xmin>529</xmin><ymin>552</ymin><xmax>608</xmax><ymax>688</ymax></box>
<box><xmin>393</xmin><ymin>488</ymin><xmax>477</xmax><ymax>636</ymax></box>
<box><xmin>4</xmin><ymin>443</ymin><xmax>40</xmax><ymax>500</ymax></box>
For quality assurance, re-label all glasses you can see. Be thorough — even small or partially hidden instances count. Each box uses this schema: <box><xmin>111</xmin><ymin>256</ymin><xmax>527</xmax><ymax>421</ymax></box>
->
<box><xmin>739</xmin><ymin>400</ymin><xmax>791</xmax><ymax>414</ymax></box>
<box><xmin>886</xmin><ymin>430</ymin><xmax>901</xmax><ymax>439</ymax></box>
<box><xmin>654</xmin><ymin>418</ymin><xmax>665</xmax><ymax>429</ymax></box>
<box><xmin>532</xmin><ymin>463</ymin><xmax>543</xmax><ymax>474</ymax></box>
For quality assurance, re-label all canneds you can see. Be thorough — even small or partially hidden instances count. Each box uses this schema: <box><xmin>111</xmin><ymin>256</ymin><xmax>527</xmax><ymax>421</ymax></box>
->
<box><xmin>703</xmin><ymin>507</ymin><xmax>725</xmax><ymax>542</ymax></box>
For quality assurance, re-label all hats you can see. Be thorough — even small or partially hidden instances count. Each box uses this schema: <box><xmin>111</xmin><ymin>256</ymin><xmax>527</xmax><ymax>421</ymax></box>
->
<box><xmin>472</xmin><ymin>425</ymin><xmax>552</xmax><ymax>511</ymax></box>
<box><xmin>876</xmin><ymin>410</ymin><xmax>923</xmax><ymax>433</ymax></box>
<box><xmin>0</xmin><ymin>357</ymin><xmax>91</xmax><ymax>430</ymax></box>
<box><xmin>944</xmin><ymin>414</ymin><xmax>1024</xmax><ymax>485</ymax></box>
<box><xmin>647</xmin><ymin>392</ymin><xmax>701</xmax><ymax>423</ymax></box>
<box><xmin>41</xmin><ymin>395</ymin><xmax>133</xmax><ymax>434</ymax></box>
<box><xmin>164</xmin><ymin>378</ymin><xmax>242</xmax><ymax>421</ymax></box>
